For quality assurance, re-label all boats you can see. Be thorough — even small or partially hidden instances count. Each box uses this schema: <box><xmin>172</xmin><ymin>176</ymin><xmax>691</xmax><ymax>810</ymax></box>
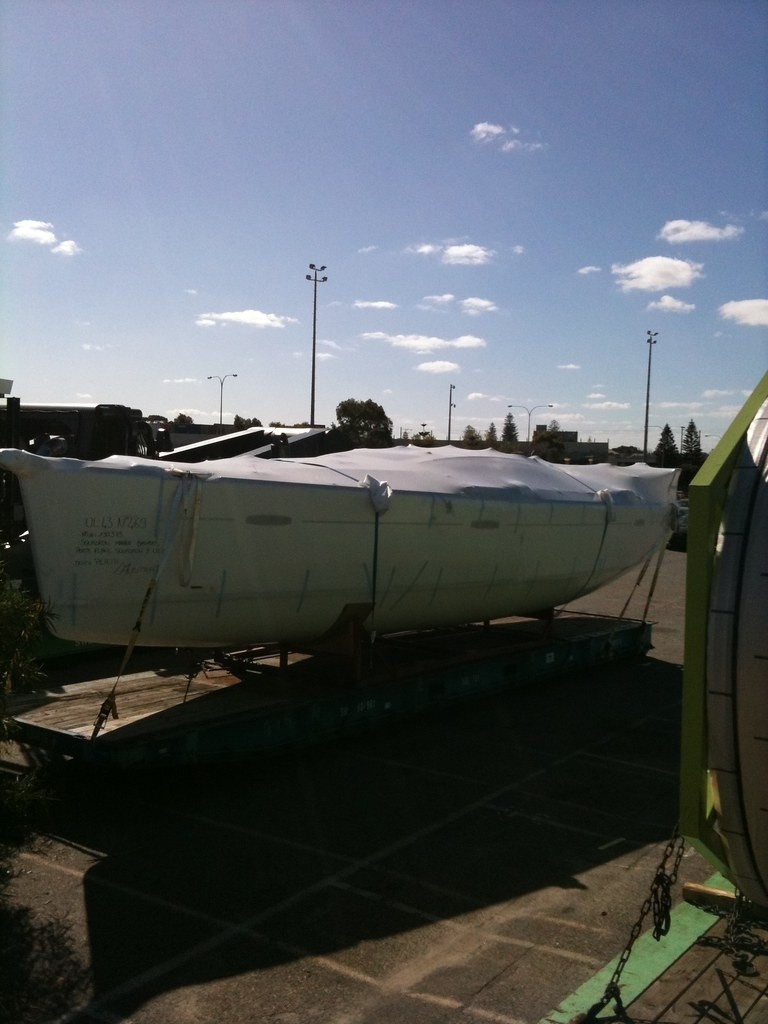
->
<box><xmin>0</xmin><ymin>437</ymin><xmax>683</xmax><ymax>649</ymax></box>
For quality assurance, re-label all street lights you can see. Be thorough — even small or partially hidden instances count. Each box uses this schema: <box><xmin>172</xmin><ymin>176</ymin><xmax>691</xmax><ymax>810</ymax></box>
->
<box><xmin>206</xmin><ymin>374</ymin><xmax>238</xmax><ymax>435</ymax></box>
<box><xmin>644</xmin><ymin>330</ymin><xmax>659</xmax><ymax>465</ymax></box>
<box><xmin>506</xmin><ymin>404</ymin><xmax>555</xmax><ymax>443</ymax></box>
<box><xmin>305</xmin><ymin>263</ymin><xmax>330</xmax><ymax>427</ymax></box>
